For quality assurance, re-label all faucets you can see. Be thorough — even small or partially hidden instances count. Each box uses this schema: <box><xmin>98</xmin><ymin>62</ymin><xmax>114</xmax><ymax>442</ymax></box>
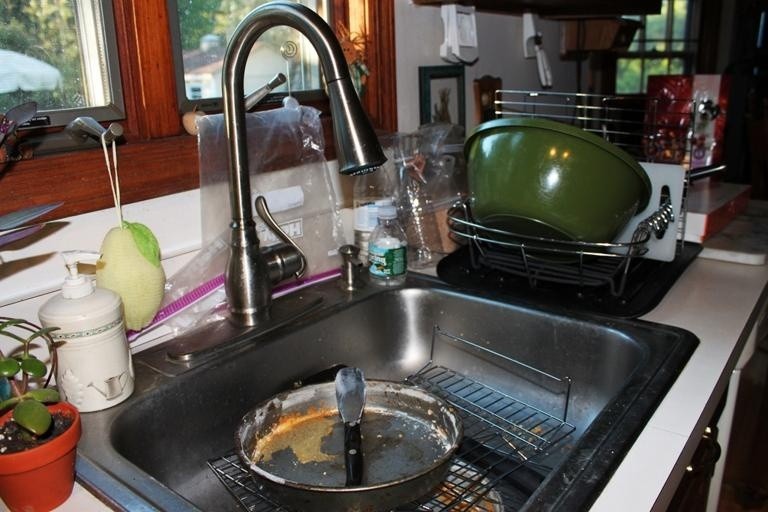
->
<box><xmin>221</xmin><ymin>1</ymin><xmax>387</xmax><ymax>220</ymax></box>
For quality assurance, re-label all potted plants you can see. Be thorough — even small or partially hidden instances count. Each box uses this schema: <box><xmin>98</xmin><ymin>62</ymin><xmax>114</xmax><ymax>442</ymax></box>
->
<box><xmin>0</xmin><ymin>320</ymin><xmax>82</xmax><ymax>512</ymax></box>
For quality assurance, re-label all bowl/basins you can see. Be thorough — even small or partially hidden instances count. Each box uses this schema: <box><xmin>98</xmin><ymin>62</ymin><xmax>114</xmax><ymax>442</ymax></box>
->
<box><xmin>231</xmin><ymin>380</ymin><xmax>461</xmax><ymax>512</ymax></box>
<box><xmin>460</xmin><ymin>116</ymin><xmax>654</xmax><ymax>269</ymax></box>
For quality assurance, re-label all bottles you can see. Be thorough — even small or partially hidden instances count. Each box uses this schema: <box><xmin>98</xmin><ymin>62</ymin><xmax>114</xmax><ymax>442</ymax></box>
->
<box><xmin>352</xmin><ymin>163</ymin><xmax>444</xmax><ymax>287</ymax></box>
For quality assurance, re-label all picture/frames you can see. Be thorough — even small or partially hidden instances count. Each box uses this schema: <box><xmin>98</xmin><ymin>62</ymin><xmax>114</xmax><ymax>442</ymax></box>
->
<box><xmin>418</xmin><ymin>65</ymin><xmax>465</xmax><ymax>138</ymax></box>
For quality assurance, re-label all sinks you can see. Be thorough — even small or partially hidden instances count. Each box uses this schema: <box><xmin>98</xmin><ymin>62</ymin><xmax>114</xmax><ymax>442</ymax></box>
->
<box><xmin>71</xmin><ymin>271</ymin><xmax>698</xmax><ymax>511</ymax></box>
